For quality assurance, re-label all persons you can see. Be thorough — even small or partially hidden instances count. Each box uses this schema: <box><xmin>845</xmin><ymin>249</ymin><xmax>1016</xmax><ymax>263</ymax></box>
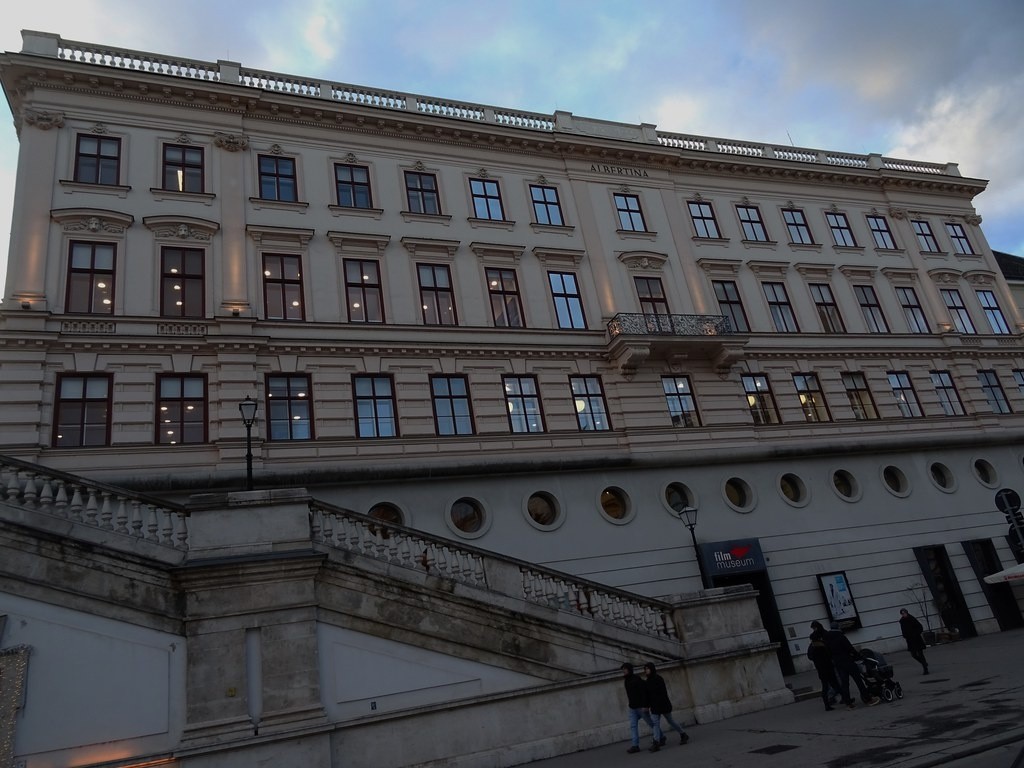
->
<box><xmin>807</xmin><ymin>619</ymin><xmax>880</xmax><ymax>712</ymax></box>
<box><xmin>643</xmin><ymin>662</ymin><xmax>690</xmax><ymax>751</ymax></box>
<box><xmin>898</xmin><ymin>608</ymin><xmax>930</xmax><ymax>675</ymax></box>
<box><xmin>621</xmin><ymin>663</ymin><xmax>667</xmax><ymax>753</ymax></box>
<box><xmin>828</xmin><ymin>584</ymin><xmax>844</xmax><ymax>615</ymax></box>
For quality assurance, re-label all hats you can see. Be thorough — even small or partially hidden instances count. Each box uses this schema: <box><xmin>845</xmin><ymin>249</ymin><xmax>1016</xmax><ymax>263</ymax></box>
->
<box><xmin>830</xmin><ymin>622</ymin><xmax>840</xmax><ymax>628</ymax></box>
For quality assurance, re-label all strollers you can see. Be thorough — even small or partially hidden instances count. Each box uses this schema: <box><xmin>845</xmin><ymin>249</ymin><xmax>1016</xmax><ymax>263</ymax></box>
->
<box><xmin>855</xmin><ymin>651</ymin><xmax>904</xmax><ymax>704</ymax></box>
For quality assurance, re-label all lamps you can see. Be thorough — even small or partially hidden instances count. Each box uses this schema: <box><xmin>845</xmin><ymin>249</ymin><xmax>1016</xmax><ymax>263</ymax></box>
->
<box><xmin>243</xmin><ymin>626</ymin><xmax>263</xmax><ymax>736</ymax></box>
<box><xmin>232</xmin><ymin>308</ymin><xmax>241</xmax><ymax>317</ymax></box>
<box><xmin>21</xmin><ymin>301</ymin><xmax>32</xmax><ymax>310</ymax></box>
<box><xmin>948</xmin><ymin>328</ymin><xmax>954</xmax><ymax>332</ymax></box>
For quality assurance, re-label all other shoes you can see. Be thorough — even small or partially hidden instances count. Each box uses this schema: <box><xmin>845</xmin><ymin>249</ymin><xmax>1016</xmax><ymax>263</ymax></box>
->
<box><xmin>851</xmin><ymin>697</ymin><xmax>856</xmax><ymax>703</ymax></box>
<box><xmin>839</xmin><ymin>698</ymin><xmax>845</xmax><ymax>704</ymax></box>
<box><xmin>923</xmin><ymin>670</ymin><xmax>929</xmax><ymax>675</ymax></box>
<box><xmin>825</xmin><ymin>706</ymin><xmax>835</xmax><ymax>711</ymax></box>
<box><xmin>649</xmin><ymin>744</ymin><xmax>660</xmax><ymax>752</ymax></box>
<box><xmin>829</xmin><ymin>697</ymin><xmax>836</xmax><ymax>704</ymax></box>
<box><xmin>679</xmin><ymin>733</ymin><xmax>689</xmax><ymax>745</ymax></box>
<box><xmin>868</xmin><ymin>698</ymin><xmax>880</xmax><ymax>706</ymax></box>
<box><xmin>658</xmin><ymin>736</ymin><xmax>666</xmax><ymax>747</ymax></box>
<box><xmin>848</xmin><ymin>704</ymin><xmax>858</xmax><ymax>711</ymax></box>
<box><xmin>627</xmin><ymin>747</ymin><xmax>640</xmax><ymax>754</ymax></box>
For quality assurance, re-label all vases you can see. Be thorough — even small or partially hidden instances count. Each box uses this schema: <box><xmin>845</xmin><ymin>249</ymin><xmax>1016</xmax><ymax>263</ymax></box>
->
<box><xmin>938</xmin><ymin>632</ymin><xmax>949</xmax><ymax>643</ymax></box>
<box><xmin>924</xmin><ymin>632</ymin><xmax>936</xmax><ymax>645</ymax></box>
<box><xmin>950</xmin><ymin>632</ymin><xmax>961</xmax><ymax>642</ymax></box>
